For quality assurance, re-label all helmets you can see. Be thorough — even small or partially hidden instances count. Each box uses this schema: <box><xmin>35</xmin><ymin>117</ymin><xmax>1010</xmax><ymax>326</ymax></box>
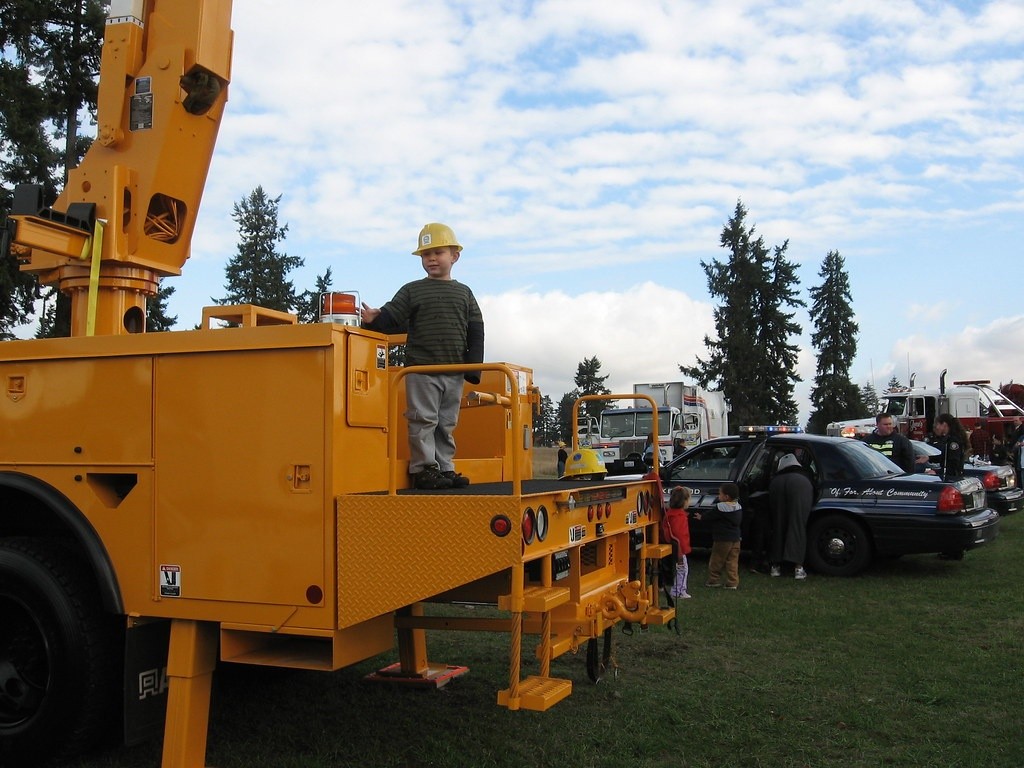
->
<box><xmin>562</xmin><ymin>449</ymin><xmax>607</xmax><ymax>478</ymax></box>
<box><xmin>559</xmin><ymin>441</ymin><xmax>566</xmax><ymax>447</ymax></box>
<box><xmin>410</xmin><ymin>223</ymin><xmax>464</xmax><ymax>255</ymax></box>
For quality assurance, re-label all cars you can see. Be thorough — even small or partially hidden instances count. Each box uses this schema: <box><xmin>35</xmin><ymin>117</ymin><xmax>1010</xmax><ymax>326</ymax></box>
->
<box><xmin>841</xmin><ymin>426</ymin><xmax>1024</xmax><ymax>516</ymax></box>
<box><xmin>605</xmin><ymin>424</ymin><xmax>1000</xmax><ymax>578</ymax></box>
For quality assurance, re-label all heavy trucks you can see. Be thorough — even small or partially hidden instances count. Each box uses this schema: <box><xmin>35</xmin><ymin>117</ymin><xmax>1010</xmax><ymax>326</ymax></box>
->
<box><xmin>577</xmin><ymin>382</ymin><xmax>728</xmax><ymax>461</ymax></box>
<box><xmin>826</xmin><ymin>369</ymin><xmax>1024</xmax><ymax>452</ymax></box>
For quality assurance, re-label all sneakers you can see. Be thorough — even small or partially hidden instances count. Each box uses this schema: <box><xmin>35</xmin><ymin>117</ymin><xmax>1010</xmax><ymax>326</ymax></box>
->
<box><xmin>771</xmin><ymin>566</ymin><xmax>780</xmax><ymax>577</ymax></box>
<box><xmin>415</xmin><ymin>467</ymin><xmax>470</xmax><ymax>490</ymax></box>
<box><xmin>794</xmin><ymin>566</ymin><xmax>807</xmax><ymax>580</ymax></box>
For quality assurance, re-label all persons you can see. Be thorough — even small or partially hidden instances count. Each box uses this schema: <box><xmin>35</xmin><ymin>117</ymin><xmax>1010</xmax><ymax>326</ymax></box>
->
<box><xmin>644</xmin><ymin>432</ymin><xmax>665</xmax><ymax>468</ymax></box>
<box><xmin>673</xmin><ymin>439</ymin><xmax>687</xmax><ymax>459</ymax></box>
<box><xmin>915</xmin><ymin>413</ymin><xmax>1024</xmax><ymax>490</ymax></box>
<box><xmin>662</xmin><ymin>486</ymin><xmax>691</xmax><ymax>598</ymax></box>
<box><xmin>356</xmin><ymin>223</ymin><xmax>484</xmax><ymax>488</ymax></box>
<box><xmin>768</xmin><ymin>465</ymin><xmax>818</xmax><ymax>580</ymax></box>
<box><xmin>860</xmin><ymin>413</ymin><xmax>914</xmax><ymax>474</ymax></box>
<box><xmin>557</xmin><ymin>442</ymin><xmax>568</xmax><ymax>478</ymax></box>
<box><xmin>693</xmin><ymin>483</ymin><xmax>742</xmax><ymax>589</ymax></box>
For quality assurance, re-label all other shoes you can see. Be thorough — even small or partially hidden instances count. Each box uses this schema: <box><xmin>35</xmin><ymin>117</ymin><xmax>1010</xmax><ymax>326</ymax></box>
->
<box><xmin>940</xmin><ymin>551</ymin><xmax>963</xmax><ymax>561</ymax></box>
<box><xmin>722</xmin><ymin>584</ymin><xmax>736</xmax><ymax>589</ymax></box>
<box><xmin>705</xmin><ymin>582</ymin><xmax>720</xmax><ymax>587</ymax></box>
<box><xmin>676</xmin><ymin>593</ymin><xmax>691</xmax><ymax>599</ymax></box>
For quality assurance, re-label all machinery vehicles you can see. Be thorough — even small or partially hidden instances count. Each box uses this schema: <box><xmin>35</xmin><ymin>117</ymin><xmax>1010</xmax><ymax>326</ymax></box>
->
<box><xmin>1</xmin><ymin>0</ymin><xmax>677</xmax><ymax>768</ymax></box>
